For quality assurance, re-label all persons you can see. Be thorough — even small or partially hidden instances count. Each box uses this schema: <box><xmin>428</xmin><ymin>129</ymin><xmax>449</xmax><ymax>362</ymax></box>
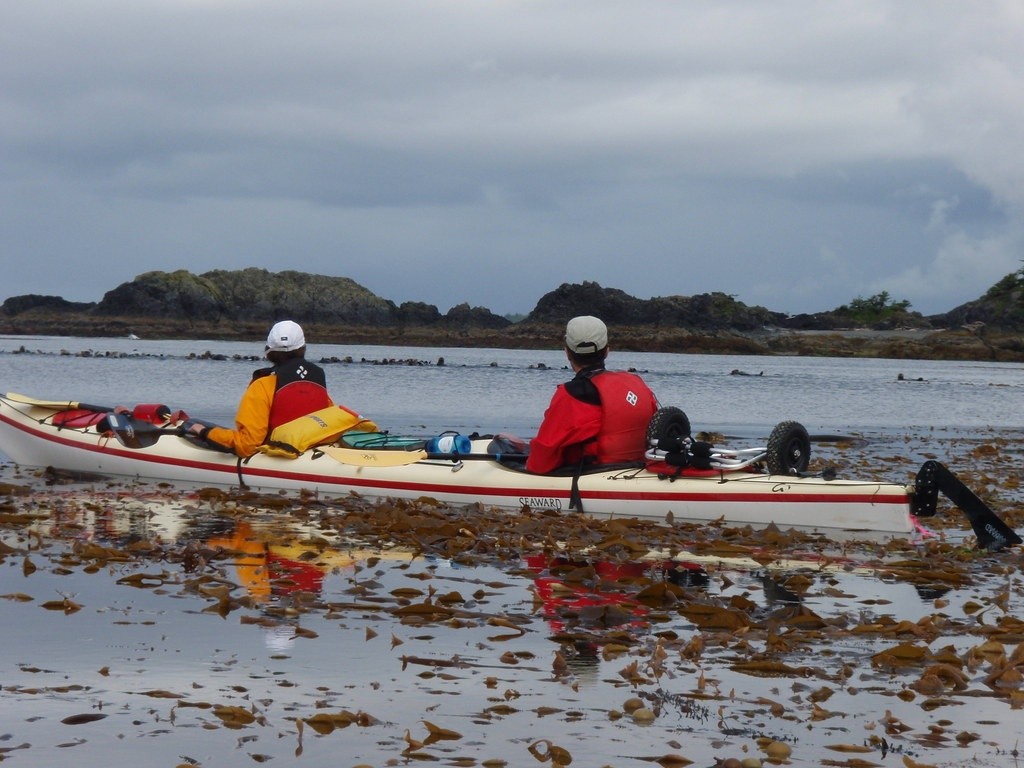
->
<box><xmin>183</xmin><ymin>319</ymin><xmax>337</xmax><ymax>459</ymax></box>
<box><xmin>524</xmin><ymin>312</ymin><xmax>660</xmax><ymax>477</ymax></box>
<box><xmin>185</xmin><ymin>495</ymin><xmax>344</xmax><ymax>659</ymax></box>
<box><xmin>525</xmin><ymin>545</ymin><xmax>712</xmax><ymax>694</ymax></box>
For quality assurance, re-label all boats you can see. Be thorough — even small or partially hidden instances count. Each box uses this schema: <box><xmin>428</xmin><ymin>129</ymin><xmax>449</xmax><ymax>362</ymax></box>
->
<box><xmin>0</xmin><ymin>391</ymin><xmax>1024</xmax><ymax>553</ymax></box>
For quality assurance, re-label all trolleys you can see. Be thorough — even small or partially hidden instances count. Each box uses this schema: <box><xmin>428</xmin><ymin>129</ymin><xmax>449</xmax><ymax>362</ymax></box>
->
<box><xmin>644</xmin><ymin>404</ymin><xmax>812</xmax><ymax>478</ymax></box>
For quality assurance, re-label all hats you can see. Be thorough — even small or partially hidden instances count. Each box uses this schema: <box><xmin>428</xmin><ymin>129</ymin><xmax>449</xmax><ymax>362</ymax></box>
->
<box><xmin>264</xmin><ymin>319</ymin><xmax>306</xmax><ymax>353</ymax></box>
<box><xmin>566</xmin><ymin>316</ymin><xmax>607</xmax><ymax>353</ymax></box>
<box><xmin>265</xmin><ymin>626</ymin><xmax>294</xmax><ymax>650</ymax></box>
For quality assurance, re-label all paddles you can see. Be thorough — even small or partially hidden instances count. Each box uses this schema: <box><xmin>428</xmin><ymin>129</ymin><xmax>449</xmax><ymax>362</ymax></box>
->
<box><xmin>106</xmin><ymin>412</ymin><xmax>200</xmax><ymax>448</ymax></box>
<box><xmin>419</xmin><ymin>451</ymin><xmax>528</xmax><ymax>462</ymax></box>
